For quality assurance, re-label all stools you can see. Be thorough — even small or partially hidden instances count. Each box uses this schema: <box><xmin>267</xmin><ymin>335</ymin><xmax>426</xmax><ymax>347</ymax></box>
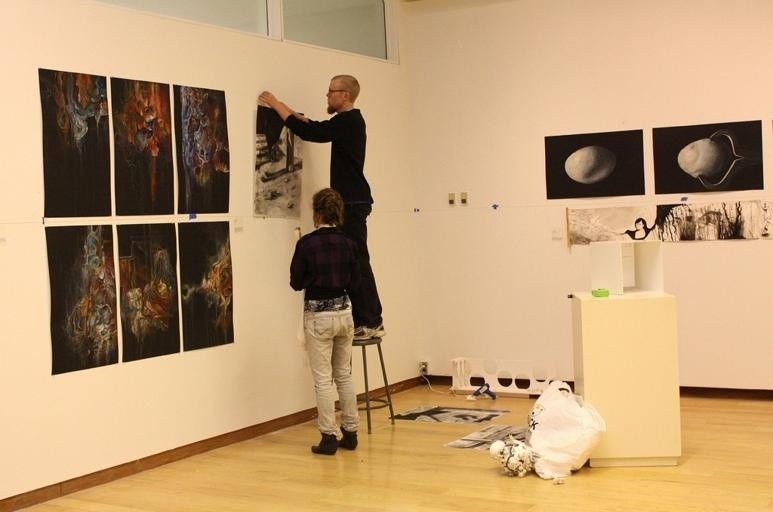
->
<box><xmin>335</xmin><ymin>337</ymin><xmax>395</xmax><ymax>434</ymax></box>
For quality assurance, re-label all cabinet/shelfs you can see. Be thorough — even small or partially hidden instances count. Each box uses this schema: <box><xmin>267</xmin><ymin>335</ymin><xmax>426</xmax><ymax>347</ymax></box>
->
<box><xmin>570</xmin><ymin>293</ymin><xmax>681</xmax><ymax>467</ymax></box>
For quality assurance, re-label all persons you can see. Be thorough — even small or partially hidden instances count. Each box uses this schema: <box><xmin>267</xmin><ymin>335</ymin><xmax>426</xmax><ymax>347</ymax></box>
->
<box><xmin>258</xmin><ymin>74</ymin><xmax>386</xmax><ymax>340</ymax></box>
<box><xmin>624</xmin><ymin>218</ymin><xmax>650</xmax><ymax>240</ymax></box>
<box><xmin>289</xmin><ymin>188</ymin><xmax>362</xmax><ymax>455</ymax></box>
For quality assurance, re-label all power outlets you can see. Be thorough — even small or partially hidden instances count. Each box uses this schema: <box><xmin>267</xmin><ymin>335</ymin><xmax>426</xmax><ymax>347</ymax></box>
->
<box><xmin>418</xmin><ymin>362</ymin><xmax>428</xmax><ymax>375</ymax></box>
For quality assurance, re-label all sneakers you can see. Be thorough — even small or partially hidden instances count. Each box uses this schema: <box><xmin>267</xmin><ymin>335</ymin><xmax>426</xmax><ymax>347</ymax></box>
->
<box><xmin>353</xmin><ymin>323</ymin><xmax>387</xmax><ymax>341</ymax></box>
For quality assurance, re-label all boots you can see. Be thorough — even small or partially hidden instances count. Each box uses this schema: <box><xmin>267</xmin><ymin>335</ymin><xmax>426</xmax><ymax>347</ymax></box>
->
<box><xmin>337</xmin><ymin>426</ymin><xmax>359</xmax><ymax>450</ymax></box>
<box><xmin>311</xmin><ymin>433</ymin><xmax>337</xmax><ymax>456</ymax></box>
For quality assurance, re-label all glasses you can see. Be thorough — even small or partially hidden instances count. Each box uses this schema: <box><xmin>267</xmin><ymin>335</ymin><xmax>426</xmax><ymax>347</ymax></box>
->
<box><xmin>329</xmin><ymin>87</ymin><xmax>346</xmax><ymax>93</ymax></box>
